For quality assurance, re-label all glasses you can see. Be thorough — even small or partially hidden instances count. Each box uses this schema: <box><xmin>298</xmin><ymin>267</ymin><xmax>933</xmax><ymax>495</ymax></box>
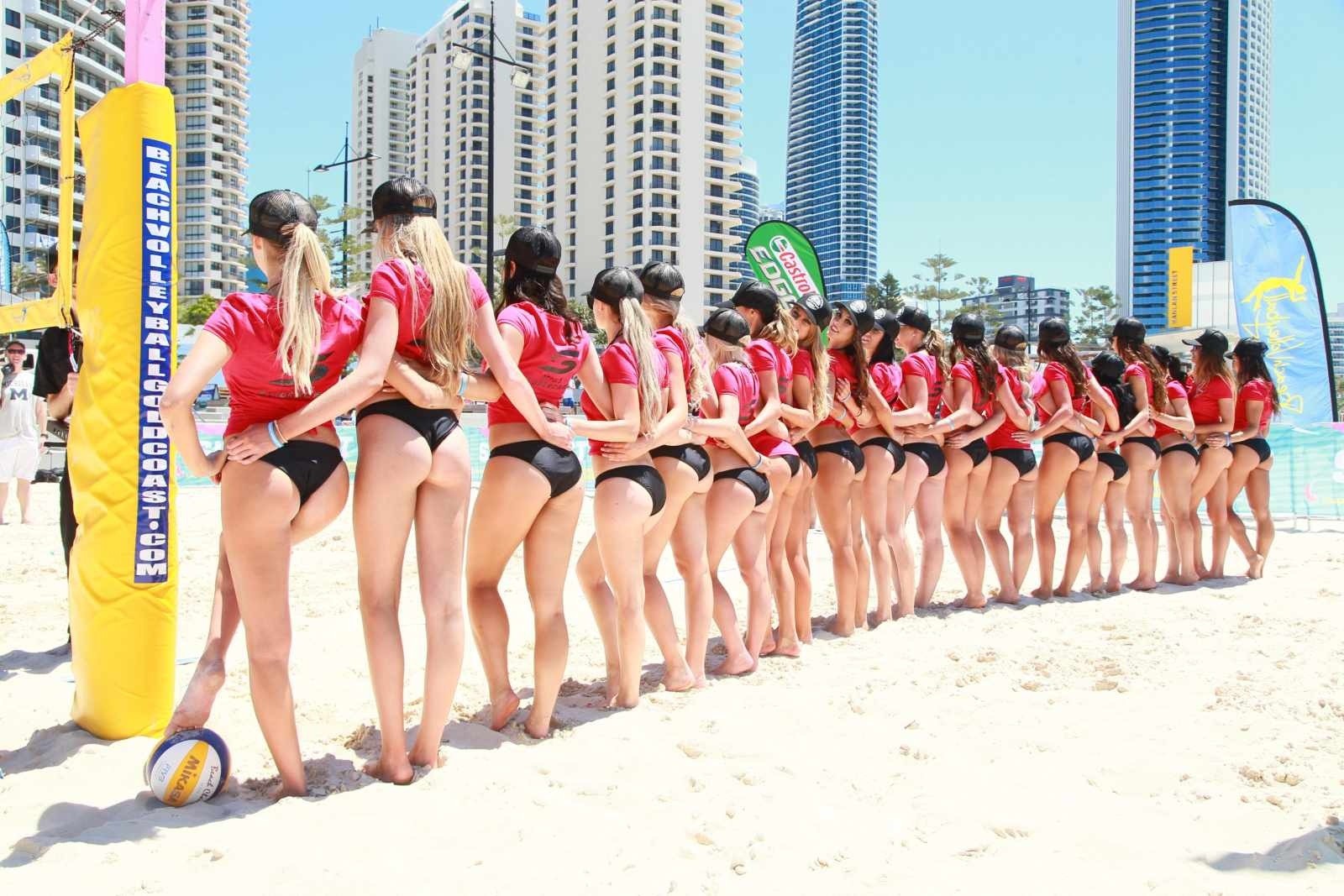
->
<box><xmin>7</xmin><ymin>349</ymin><xmax>25</xmax><ymax>354</ymax></box>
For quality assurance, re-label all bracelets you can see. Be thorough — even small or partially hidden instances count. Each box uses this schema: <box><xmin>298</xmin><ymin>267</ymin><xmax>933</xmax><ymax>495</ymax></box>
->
<box><xmin>268</xmin><ymin>422</ymin><xmax>283</xmax><ymax>448</ymax></box>
<box><xmin>39</xmin><ymin>433</ymin><xmax>48</xmax><ymax>439</ymax></box>
<box><xmin>751</xmin><ymin>454</ymin><xmax>762</xmax><ymax>469</ymax></box>
<box><xmin>565</xmin><ymin>416</ymin><xmax>571</xmax><ymax>429</ymax></box>
<box><xmin>944</xmin><ymin>417</ymin><xmax>955</xmax><ymax>431</ymax></box>
<box><xmin>457</xmin><ymin>373</ymin><xmax>467</xmax><ymax>395</ymax></box>
<box><xmin>274</xmin><ymin>419</ymin><xmax>287</xmax><ymax>443</ymax></box>
<box><xmin>834</xmin><ymin>408</ymin><xmax>846</xmax><ymax>421</ymax></box>
<box><xmin>1225</xmin><ymin>432</ymin><xmax>1229</xmax><ymax>446</ymax></box>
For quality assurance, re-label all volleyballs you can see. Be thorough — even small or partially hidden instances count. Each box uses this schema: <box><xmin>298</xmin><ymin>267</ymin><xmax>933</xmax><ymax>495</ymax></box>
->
<box><xmin>147</xmin><ymin>728</ymin><xmax>232</xmax><ymax>806</ymax></box>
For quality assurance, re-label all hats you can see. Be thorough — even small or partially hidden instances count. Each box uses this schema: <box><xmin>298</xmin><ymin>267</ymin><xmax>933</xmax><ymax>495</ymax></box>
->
<box><xmin>947</xmin><ymin>314</ymin><xmax>985</xmax><ymax>340</ymax></box>
<box><xmin>241</xmin><ymin>190</ymin><xmax>320</xmax><ymax>245</ymax></box>
<box><xmin>1182</xmin><ymin>329</ymin><xmax>1228</xmax><ymax>351</ymax></box>
<box><xmin>361</xmin><ymin>174</ymin><xmax>437</xmax><ymax>234</ymax></box>
<box><xmin>995</xmin><ymin>324</ymin><xmax>1028</xmax><ymax>352</ymax></box>
<box><xmin>1224</xmin><ymin>337</ymin><xmax>1268</xmax><ymax>360</ymax></box>
<box><xmin>1084</xmin><ymin>351</ymin><xmax>1123</xmax><ymax>379</ymax></box>
<box><xmin>580</xmin><ymin>265</ymin><xmax>644</xmax><ymax>308</ymax></box>
<box><xmin>47</xmin><ymin>239</ymin><xmax>79</xmax><ymax>271</ymax></box>
<box><xmin>1102</xmin><ymin>316</ymin><xmax>1145</xmax><ymax>343</ymax></box>
<box><xmin>639</xmin><ymin>261</ymin><xmax>685</xmax><ymax>301</ymax></box>
<box><xmin>491</xmin><ymin>225</ymin><xmax>562</xmax><ymax>275</ymax></box>
<box><xmin>1039</xmin><ymin>318</ymin><xmax>1070</xmax><ymax>343</ymax></box>
<box><xmin>698</xmin><ymin>282</ymin><xmax>932</xmax><ymax>347</ymax></box>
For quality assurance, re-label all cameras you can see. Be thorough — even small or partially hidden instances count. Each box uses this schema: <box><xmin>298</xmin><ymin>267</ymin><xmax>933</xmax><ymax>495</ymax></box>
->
<box><xmin>2</xmin><ymin>363</ymin><xmax>14</xmax><ymax>376</ymax></box>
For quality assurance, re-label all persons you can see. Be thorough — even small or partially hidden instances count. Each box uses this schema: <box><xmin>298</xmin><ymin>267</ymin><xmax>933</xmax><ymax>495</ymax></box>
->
<box><xmin>157</xmin><ymin>178</ymin><xmax>1281</xmax><ymax>802</ymax></box>
<box><xmin>0</xmin><ymin>340</ymin><xmax>49</xmax><ymax>525</ymax></box>
<box><xmin>31</xmin><ymin>239</ymin><xmax>83</xmax><ymax>651</ymax></box>
